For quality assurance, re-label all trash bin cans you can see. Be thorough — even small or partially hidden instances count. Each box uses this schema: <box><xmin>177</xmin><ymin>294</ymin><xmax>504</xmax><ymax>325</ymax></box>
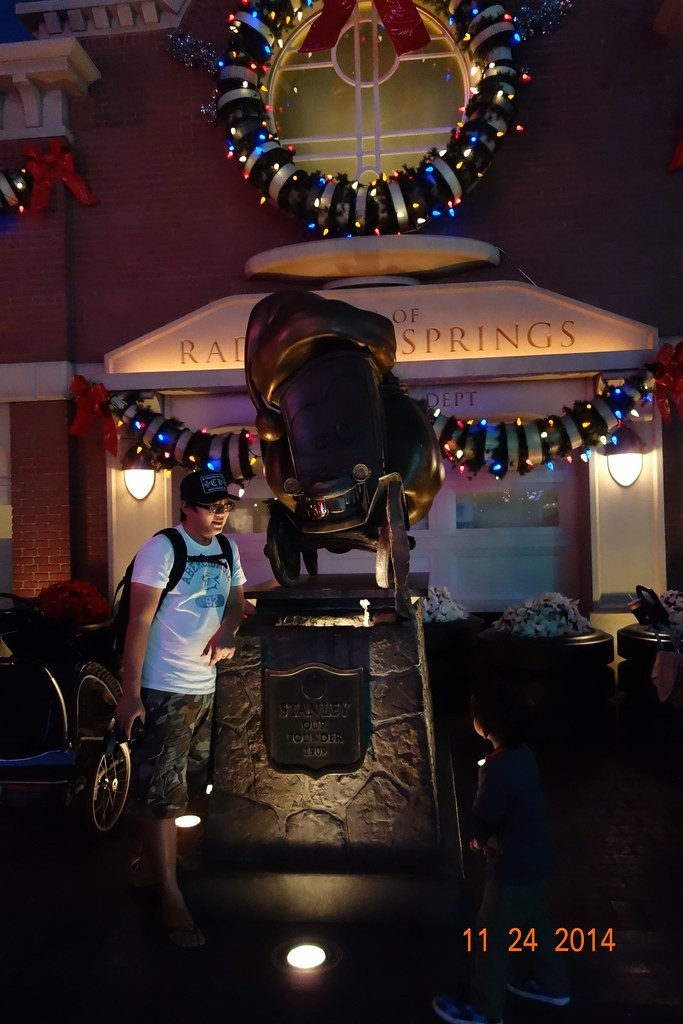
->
<box><xmin>615</xmin><ymin>622</ymin><xmax>682</xmax><ymax>742</ymax></box>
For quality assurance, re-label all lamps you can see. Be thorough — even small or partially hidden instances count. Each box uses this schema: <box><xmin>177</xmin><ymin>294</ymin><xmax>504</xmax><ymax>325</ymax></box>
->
<box><xmin>120</xmin><ymin>443</ymin><xmax>155</xmax><ymax>501</ymax></box>
<box><xmin>262</xmin><ymin>929</ymin><xmax>348</xmax><ymax>996</ymax></box>
<box><xmin>598</xmin><ymin>424</ymin><xmax>647</xmax><ymax>489</ymax></box>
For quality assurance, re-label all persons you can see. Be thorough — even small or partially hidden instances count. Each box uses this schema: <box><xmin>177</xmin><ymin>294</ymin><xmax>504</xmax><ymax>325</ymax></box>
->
<box><xmin>114</xmin><ymin>470</ymin><xmax>256</xmax><ymax>952</ymax></box>
<box><xmin>431</xmin><ymin>683</ymin><xmax>571</xmax><ymax>1024</ymax></box>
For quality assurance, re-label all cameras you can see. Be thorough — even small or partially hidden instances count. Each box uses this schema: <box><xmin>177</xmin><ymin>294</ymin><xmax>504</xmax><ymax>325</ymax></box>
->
<box><xmin>110</xmin><ymin>714</ymin><xmax>153</xmax><ymax>749</ymax></box>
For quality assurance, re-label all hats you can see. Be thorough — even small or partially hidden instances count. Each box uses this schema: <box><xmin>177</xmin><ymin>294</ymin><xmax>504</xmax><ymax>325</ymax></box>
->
<box><xmin>180</xmin><ymin>470</ymin><xmax>240</xmax><ymax>504</ymax></box>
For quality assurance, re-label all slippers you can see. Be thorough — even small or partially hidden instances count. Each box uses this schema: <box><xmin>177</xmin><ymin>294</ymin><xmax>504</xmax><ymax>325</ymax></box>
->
<box><xmin>155</xmin><ymin>899</ymin><xmax>208</xmax><ymax>956</ymax></box>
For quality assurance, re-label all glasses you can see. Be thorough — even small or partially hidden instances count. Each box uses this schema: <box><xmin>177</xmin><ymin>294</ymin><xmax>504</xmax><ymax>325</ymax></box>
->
<box><xmin>193</xmin><ymin>502</ymin><xmax>235</xmax><ymax>513</ymax></box>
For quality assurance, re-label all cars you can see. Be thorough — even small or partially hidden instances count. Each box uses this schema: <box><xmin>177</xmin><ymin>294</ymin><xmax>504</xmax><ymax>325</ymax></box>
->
<box><xmin>0</xmin><ymin>591</ymin><xmax>130</xmax><ymax>834</ymax></box>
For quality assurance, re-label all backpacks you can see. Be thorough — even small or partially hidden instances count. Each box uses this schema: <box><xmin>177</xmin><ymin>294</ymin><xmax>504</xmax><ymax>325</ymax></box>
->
<box><xmin>110</xmin><ymin>528</ymin><xmax>233</xmax><ymax>657</ymax></box>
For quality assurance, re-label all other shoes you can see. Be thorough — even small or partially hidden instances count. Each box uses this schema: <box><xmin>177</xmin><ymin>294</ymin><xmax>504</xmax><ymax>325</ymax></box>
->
<box><xmin>505</xmin><ymin>966</ymin><xmax>571</xmax><ymax>1007</ymax></box>
<box><xmin>433</xmin><ymin>995</ymin><xmax>503</xmax><ymax>1024</ymax></box>
